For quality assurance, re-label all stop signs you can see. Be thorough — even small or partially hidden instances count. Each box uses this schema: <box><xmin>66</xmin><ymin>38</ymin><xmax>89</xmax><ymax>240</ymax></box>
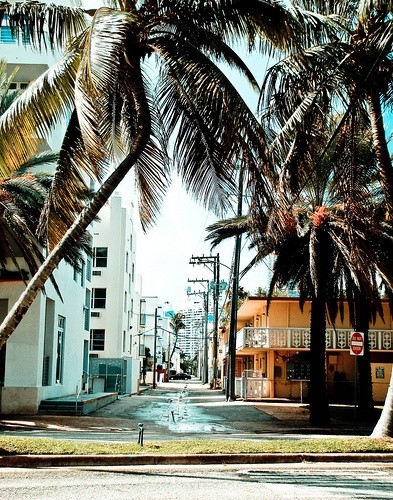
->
<box><xmin>350</xmin><ymin>332</ymin><xmax>365</xmax><ymax>356</ymax></box>
